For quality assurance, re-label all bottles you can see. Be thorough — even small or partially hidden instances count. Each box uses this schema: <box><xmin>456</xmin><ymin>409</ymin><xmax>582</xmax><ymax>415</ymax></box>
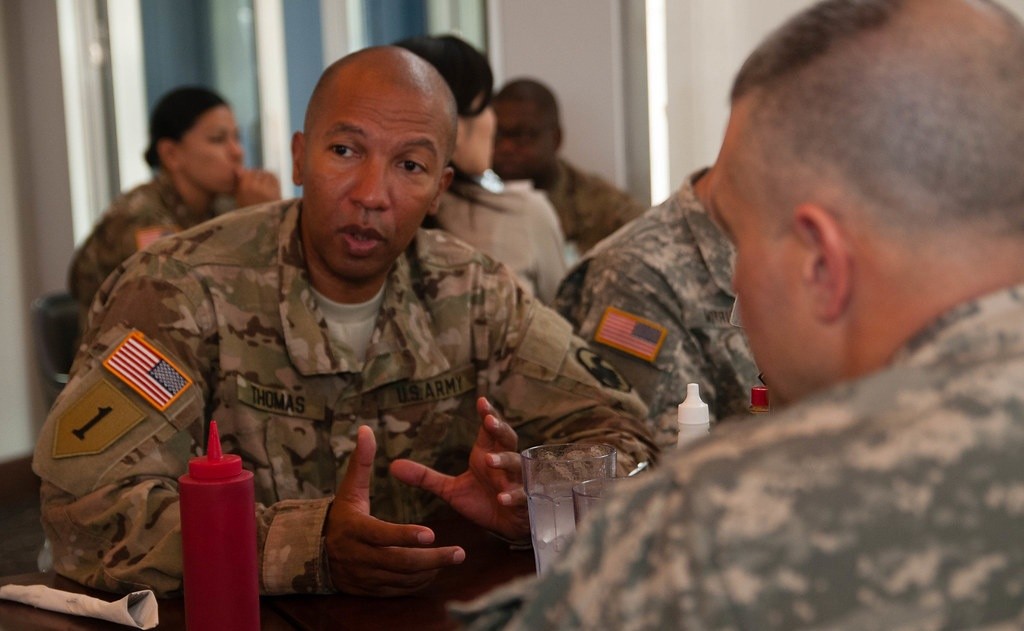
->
<box><xmin>747</xmin><ymin>387</ymin><xmax>770</xmax><ymax>415</ymax></box>
<box><xmin>677</xmin><ymin>383</ymin><xmax>711</xmax><ymax>452</ymax></box>
<box><xmin>178</xmin><ymin>421</ymin><xmax>260</xmax><ymax>631</ymax></box>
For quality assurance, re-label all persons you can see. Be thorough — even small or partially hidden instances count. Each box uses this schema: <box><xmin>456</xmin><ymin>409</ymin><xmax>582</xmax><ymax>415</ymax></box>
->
<box><xmin>31</xmin><ymin>44</ymin><xmax>667</xmax><ymax>597</ymax></box>
<box><xmin>498</xmin><ymin>0</ymin><xmax>1024</xmax><ymax>630</ymax></box>
<box><xmin>491</xmin><ymin>77</ymin><xmax>646</xmax><ymax>258</ymax></box>
<box><xmin>549</xmin><ymin>166</ymin><xmax>765</xmax><ymax>449</ymax></box>
<box><xmin>66</xmin><ymin>86</ymin><xmax>282</xmax><ymax>330</ymax></box>
<box><xmin>401</xmin><ymin>34</ymin><xmax>569</xmax><ymax>302</ymax></box>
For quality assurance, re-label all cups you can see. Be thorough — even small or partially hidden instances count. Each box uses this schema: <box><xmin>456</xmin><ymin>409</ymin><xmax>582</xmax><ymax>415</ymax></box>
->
<box><xmin>521</xmin><ymin>442</ymin><xmax>617</xmax><ymax>577</ymax></box>
<box><xmin>572</xmin><ymin>476</ymin><xmax>632</xmax><ymax>529</ymax></box>
